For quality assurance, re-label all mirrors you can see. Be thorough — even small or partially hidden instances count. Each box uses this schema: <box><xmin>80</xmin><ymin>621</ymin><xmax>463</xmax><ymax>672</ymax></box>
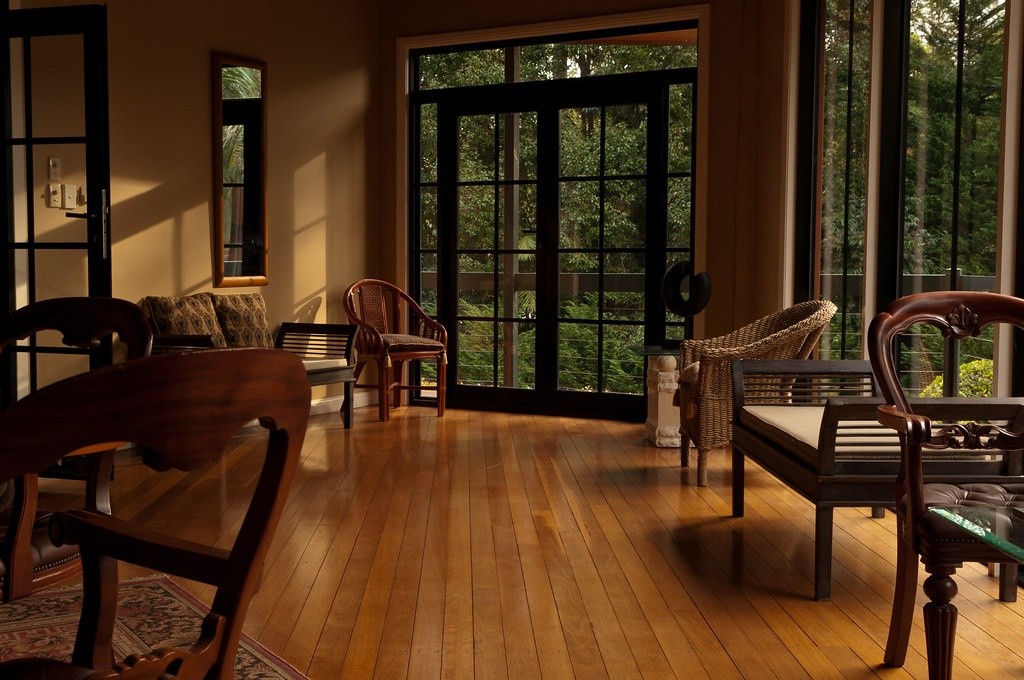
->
<box><xmin>211</xmin><ymin>51</ymin><xmax>270</xmax><ymax>286</ymax></box>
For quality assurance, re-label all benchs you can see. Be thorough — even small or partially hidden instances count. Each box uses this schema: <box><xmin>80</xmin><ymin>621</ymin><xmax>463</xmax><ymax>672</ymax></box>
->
<box><xmin>728</xmin><ymin>358</ymin><xmax>1024</xmax><ymax>602</ymax></box>
<box><xmin>150</xmin><ymin>322</ymin><xmax>360</xmax><ymax>429</ymax></box>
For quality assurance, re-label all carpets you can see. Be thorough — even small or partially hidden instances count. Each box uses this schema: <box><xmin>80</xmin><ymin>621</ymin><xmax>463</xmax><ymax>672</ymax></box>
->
<box><xmin>0</xmin><ymin>573</ymin><xmax>311</xmax><ymax>680</ymax></box>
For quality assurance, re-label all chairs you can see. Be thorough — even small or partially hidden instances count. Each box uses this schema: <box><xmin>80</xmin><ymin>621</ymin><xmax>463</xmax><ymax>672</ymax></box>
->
<box><xmin>0</xmin><ymin>347</ymin><xmax>312</xmax><ymax>680</ymax></box>
<box><xmin>340</xmin><ymin>279</ymin><xmax>448</xmax><ymax>421</ymax></box>
<box><xmin>673</xmin><ymin>300</ymin><xmax>839</xmax><ymax>487</ymax></box>
<box><xmin>868</xmin><ymin>290</ymin><xmax>1024</xmax><ymax>680</ymax></box>
<box><xmin>0</xmin><ymin>297</ymin><xmax>152</xmax><ymax>605</ymax></box>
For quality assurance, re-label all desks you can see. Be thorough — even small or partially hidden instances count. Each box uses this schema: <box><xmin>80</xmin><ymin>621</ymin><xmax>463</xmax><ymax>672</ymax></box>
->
<box><xmin>626</xmin><ymin>345</ymin><xmax>696</xmax><ymax>447</ymax></box>
<box><xmin>926</xmin><ymin>506</ymin><xmax>1024</xmax><ymax>566</ymax></box>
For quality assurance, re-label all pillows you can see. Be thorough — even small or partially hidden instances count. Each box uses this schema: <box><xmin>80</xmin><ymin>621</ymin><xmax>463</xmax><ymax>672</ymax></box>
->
<box><xmin>144</xmin><ymin>293</ymin><xmax>226</xmax><ymax>349</ymax></box>
<box><xmin>213</xmin><ymin>294</ymin><xmax>275</xmax><ymax>349</ymax></box>
